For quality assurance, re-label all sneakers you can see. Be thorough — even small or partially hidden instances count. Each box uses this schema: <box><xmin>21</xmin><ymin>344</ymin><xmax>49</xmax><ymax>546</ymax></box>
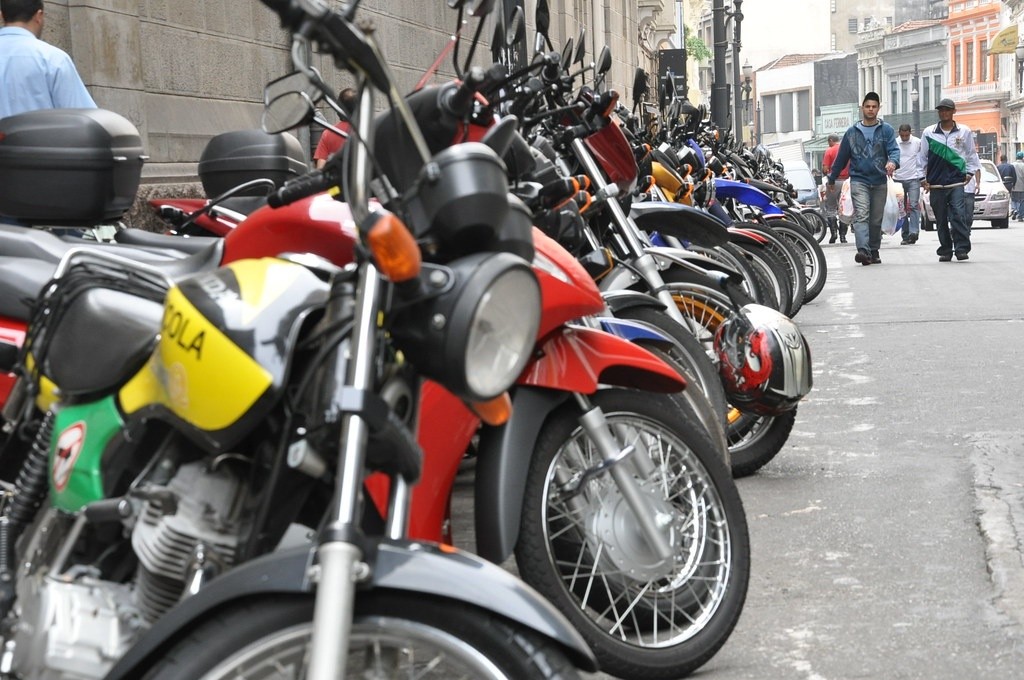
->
<box><xmin>871</xmin><ymin>249</ymin><xmax>881</xmax><ymax>262</ymax></box>
<box><xmin>855</xmin><ymin>249</ymin><xmax>872</xmax><ymax>265</ymax></box>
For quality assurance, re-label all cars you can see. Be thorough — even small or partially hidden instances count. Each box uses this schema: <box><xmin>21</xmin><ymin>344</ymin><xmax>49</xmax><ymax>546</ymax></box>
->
<box><xmin>775</xmin><ymin>159</ymin><xmax>822</xmax><ymax>208</ymax></box>
<box><xmin>919</xmin><ymin>160</ymin><xmax>1013</xmax><ymax>229</ymax></box>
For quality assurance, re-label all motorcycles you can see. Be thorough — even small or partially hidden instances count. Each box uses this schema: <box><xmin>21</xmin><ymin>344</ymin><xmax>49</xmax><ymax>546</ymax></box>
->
<box><xmin>1</xmin><ymin>0</ymin><xmax>830</xmax><ymax>680</ymax></box>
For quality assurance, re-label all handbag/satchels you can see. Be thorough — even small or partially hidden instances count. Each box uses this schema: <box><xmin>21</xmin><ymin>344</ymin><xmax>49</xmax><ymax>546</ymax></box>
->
<box><xmin>838</xmin><ymin>176</ymin><xmax>854</xmax><ymax>225</ymax></box>
<box><xmin>881</xmin><ymin>174</ymin><xmax>906</xmax><ymax>236</ymax></box>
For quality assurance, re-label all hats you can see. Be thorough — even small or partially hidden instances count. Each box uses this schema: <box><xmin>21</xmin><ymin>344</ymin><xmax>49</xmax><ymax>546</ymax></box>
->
<box><xmin>1017</xmin><ymin>151</ymin><xmax>1024</xmax><ymax>158</ymax></box>
<box><xmin>934</xmin><ymin>98</ymin><xmax>955</xmax><ymax>110</ymax></box>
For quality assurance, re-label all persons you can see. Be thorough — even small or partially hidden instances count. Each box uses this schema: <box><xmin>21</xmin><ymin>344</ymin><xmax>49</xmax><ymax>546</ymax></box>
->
<box><xmin>996</xmin><ymin>150</ymin><xmax>1024</xmax><ymax>223</ymax></box>
<box><xmin>922</xmin><ymin>100</ymin><xmax>982</xmax><ymax>262</ymax></box>
<box><xmin>826</xmin><ymin>93</ymin><xmax>900</xmax><ymax>265</ymax></box>
<box><xmin>823</xmin><ymin>133</ymin><xmax>850</xmax><ymax>243</ymax></box>
<box><xmin>883</xmin><ymin>123</ymin><xmax>928</xmax><ymax>245</ymax></box>
<box><xmin>0</xmin><ymin>0</ymin><xmax>98</xmax><ymax>120</ymax></box>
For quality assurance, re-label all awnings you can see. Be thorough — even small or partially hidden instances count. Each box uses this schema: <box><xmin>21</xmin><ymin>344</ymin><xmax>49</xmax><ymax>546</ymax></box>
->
<box><xmin>984</xmin><ymin>24</ymin><xmax>1018</xmax><ymax>56</ymax></box>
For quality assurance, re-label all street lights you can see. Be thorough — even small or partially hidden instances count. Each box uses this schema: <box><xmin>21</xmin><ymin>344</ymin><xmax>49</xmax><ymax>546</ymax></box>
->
<box><xmin>909</xmin><ymin>88</ymin><xmax>920</xmax><ymax>137</ymax></box>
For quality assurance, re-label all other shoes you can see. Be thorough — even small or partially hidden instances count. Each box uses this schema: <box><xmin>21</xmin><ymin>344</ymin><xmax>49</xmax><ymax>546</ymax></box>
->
<box><xmin>955</xmin><ymin>250</ymin><xmax>968</xmax><ymax>260</ymax></box>
<box><xmin>1012</xmin><ymin>212</ymin><xmax>1018</xmax><ymax>220</ymax></box>
<box><xmin>909</xmin><ymin>232</ymin><xmax>918</xmax><ymax>244</ymax></box>
<box><xmin>1018</xmin><ymin>218</ymin><xmax>1024</xmax><ymax>222</ymax></box>
<box><xmin>901</xmin><ymin>235</ymin><xmax>909</xmax><ymax>245</ymax></box>
<box><xmin>939</xmin><ymin>252</ymin><xmax>953</xmax><ymax>261</ymax></box>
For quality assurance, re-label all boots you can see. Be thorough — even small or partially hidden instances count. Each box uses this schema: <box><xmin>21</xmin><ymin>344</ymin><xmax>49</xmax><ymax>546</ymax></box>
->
<box><xmin>838</xmin><ymin>221</ymin><xmax>849</xmax><ymax>243</ymax></box>
<box><xmin>827</xmin><ymin>217</ymin><xmax>838</xmax><ymax>243</ymax></box>
